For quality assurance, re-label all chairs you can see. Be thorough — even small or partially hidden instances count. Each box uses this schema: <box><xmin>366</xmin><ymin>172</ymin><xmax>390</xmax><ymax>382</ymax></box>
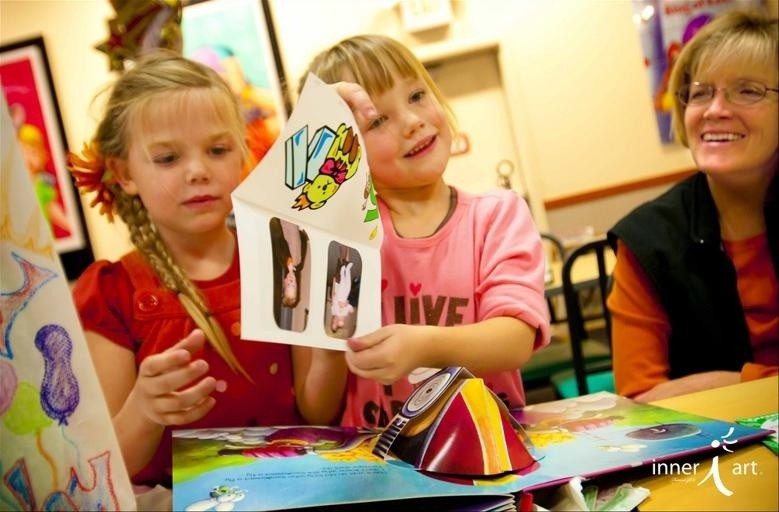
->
<box><xmin>550</xmin><ymin>239</ymin><xmax>612</xmax><ymax>400</ymax></box>
<box><xmin>541</xmin><ymin>235</ymin><xmax>563</xmax><ymax>265</ymax></box>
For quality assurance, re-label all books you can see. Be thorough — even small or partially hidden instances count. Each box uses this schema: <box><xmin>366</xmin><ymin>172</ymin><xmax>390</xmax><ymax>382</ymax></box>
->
<box><xmin>169</xmin><ymin>362</ymin><xmax>775</xmax><ymax>512</ymax></box>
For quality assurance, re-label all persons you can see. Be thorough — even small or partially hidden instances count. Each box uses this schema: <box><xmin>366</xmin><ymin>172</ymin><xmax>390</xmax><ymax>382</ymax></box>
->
<box><xmin>326</xmin><ymin>240</ymin><xmax>357</xmax><ymax>333</ymax></box>
<box><xmin>601</xmin><ymin>9</ymin><xmax>778</xmax><ymax>406</ymax></box>
<box><xmin>271</xmin><ymin>216</ymin><xmax>310</xmax><ymax>311</ymax></box>
<box><xmin>64</xmin><ymin>48</ymin><xmax>349</xmax><ymax>490</ymax></box>
<box><xmin>293</xmin><ymin>32</ymin><xmax>555</xmax><ymax>436</ymax></box>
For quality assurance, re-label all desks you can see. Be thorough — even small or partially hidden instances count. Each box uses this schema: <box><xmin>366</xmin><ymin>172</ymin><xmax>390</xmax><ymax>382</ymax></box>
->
<box><xmin>627</xmin><ymin>372</ymin><xmax>778</xmax><ymax>512</ymax></box>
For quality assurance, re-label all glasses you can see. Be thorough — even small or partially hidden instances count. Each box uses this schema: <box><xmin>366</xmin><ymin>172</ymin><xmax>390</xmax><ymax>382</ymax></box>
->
<box><xmin>674</xmin><ymin>82</ymin><xmax>779</xmax><ymax>106</ymax></box>
